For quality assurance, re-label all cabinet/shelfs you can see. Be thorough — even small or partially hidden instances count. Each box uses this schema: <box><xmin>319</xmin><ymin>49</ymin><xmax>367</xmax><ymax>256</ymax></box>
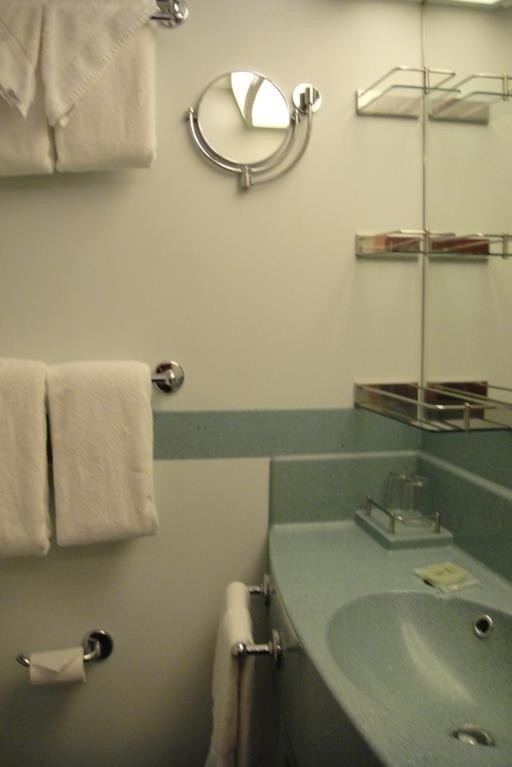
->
<box><xmin>352</xmin><ymin>63</ymin><xmax>508</xmax><ymax>429</ymax></box>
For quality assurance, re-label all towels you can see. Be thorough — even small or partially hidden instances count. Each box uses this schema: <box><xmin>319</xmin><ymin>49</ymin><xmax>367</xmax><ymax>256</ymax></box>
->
<box><xmin>0</xmin><ymin>358</ymin><xmax>162</xmax><ymax>557</ymax></box>
<box><xmin>0</xmin><ymin>0</ymin><xmax>164</xmax><ymax>175</ymax></box>
<box><xmin>205</xmin><ymin>585</ymin><xmax>256</xmax><ymax>767</ymax></box>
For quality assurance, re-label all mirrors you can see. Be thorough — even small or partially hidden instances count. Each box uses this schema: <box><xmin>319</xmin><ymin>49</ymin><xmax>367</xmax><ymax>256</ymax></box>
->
<box><xmin>423</xmin><ymin>1</ymin><xmax>512</xmax><ymax>434</ymax></box>
<box><xmin>188</xmin><ymin>71</ymin><xmax>320</xmax><ymax>189</ymax></box>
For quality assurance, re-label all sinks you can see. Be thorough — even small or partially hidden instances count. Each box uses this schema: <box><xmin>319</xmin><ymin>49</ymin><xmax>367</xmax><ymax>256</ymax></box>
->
<box><xmin>326</xmin><ymin>591</ymin><xmax>512</xmax><ymax>766</ymax></box>
